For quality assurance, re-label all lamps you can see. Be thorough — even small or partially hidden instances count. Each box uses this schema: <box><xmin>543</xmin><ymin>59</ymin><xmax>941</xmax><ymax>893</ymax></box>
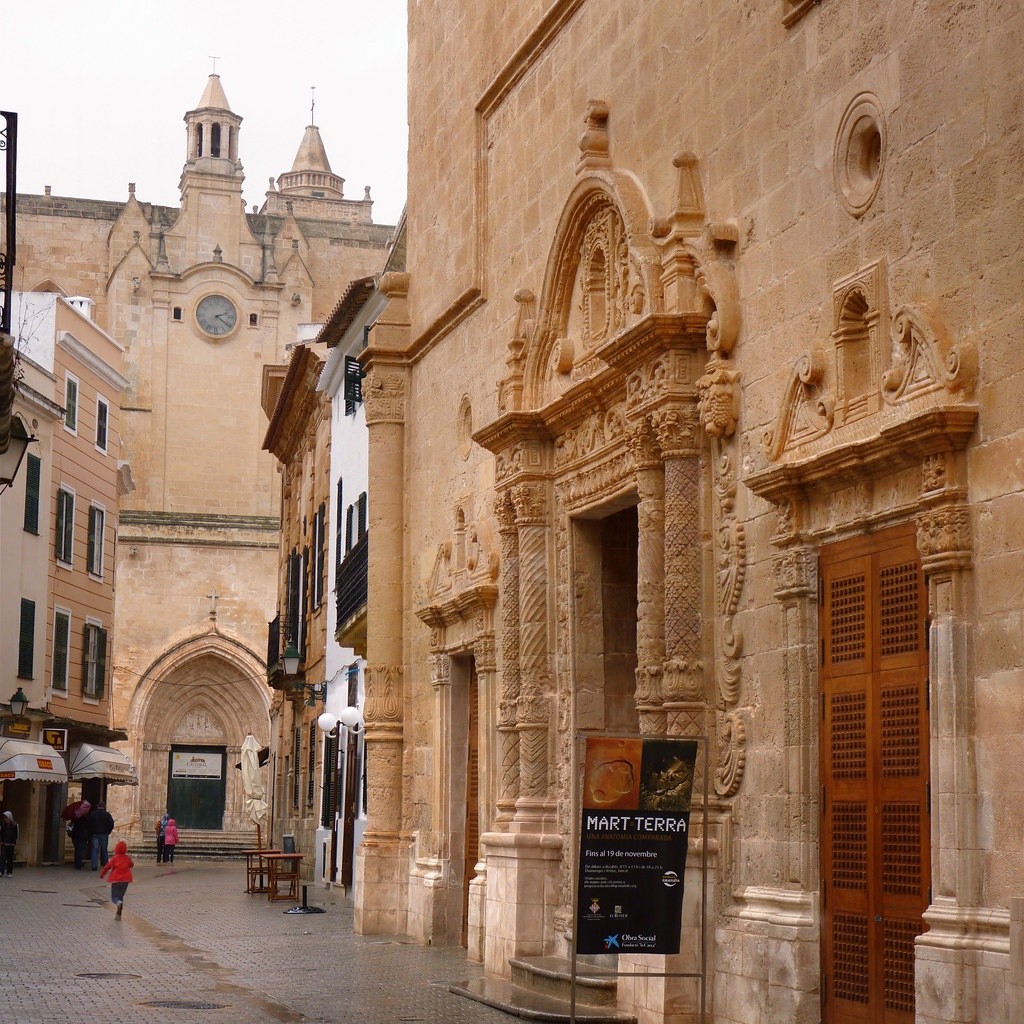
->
<box><xmin>0</xmin><ymin>687</ymin><xmax>29</xmax><ymax>728</ymax></box>
<box><xmin>303</xmin><ymin>696</ymin><xmax>315</xmax><ymax>707</ymax></box>
<box><xmin>317</xmin><ymin>713</ymin><xmax>338</xmax><ymax>739</ymax></box>
<box><xmin>0</xmin><ymin>415</ymin><xmax>40</xmax><ymax>496</ymax></box>
<box><xmin>280</xmin><ymin>640</ymin><xmax>327</xmax><ymax>702</ymax></box>
<box><xmin>341</xmin><ymin>707</ymin><xmax>365</xmax><ymax>734</ymax></box>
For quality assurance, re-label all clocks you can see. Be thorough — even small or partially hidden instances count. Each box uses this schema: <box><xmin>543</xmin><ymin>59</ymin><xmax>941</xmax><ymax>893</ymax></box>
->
<box><xmin>196</xmin><ymin>294</ymin><xmax>238</xmax><ymax>336</ymax></box>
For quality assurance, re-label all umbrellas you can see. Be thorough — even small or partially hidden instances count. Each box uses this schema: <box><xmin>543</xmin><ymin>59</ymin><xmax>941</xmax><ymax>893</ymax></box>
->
<box><xmin>240</xmin><ymin>732</ymin><xmax>269</xmax><ymax>887</ymax></box>
<box><xmin>62</xmin><ymin>799</ymin><xmax>91</xmax><ymax>821</ymax></box>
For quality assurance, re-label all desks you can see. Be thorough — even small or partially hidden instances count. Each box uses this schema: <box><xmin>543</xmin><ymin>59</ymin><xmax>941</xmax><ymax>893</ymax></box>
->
<box><xmin>260</xmin><ymin>853</ymin><xmax>307</xmax><ymax>904</ymax></box>
<box><xmin>240</xmin><ymin>849</ymin><xmax>281</xmax><ymax>896</ymax></box>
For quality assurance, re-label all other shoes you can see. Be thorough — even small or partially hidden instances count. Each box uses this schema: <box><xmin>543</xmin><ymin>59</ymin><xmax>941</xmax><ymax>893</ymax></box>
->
<box><xmin>7</xmin><ymin>873</ymin><xmax>12</xmax><ymax>878</ymax></box>
<box><xmin>0</xmin><ymin>872</ymin><xmax>2</xmax><ymax>876</ymax></box>
<box><xmin>92</xmin><ymin>868</ymin><xmax>97</xmax><ymax>871</ymax></box>
<box><xmin>114</xmin><ymin>901</ymin><xmax>123</xmax><ymax>920</ymax></box>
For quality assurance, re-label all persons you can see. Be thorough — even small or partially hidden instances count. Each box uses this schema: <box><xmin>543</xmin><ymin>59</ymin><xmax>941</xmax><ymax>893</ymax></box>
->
<box><xmin>87</xmin><ymin>799</ymin><xmax>115</xmax><ymax>872</ymax></box>
<box><xmin>99</xmin><ymin>841</ymin><xmax>135</xmax><ymax>921</ymax></box>
<box><xmin>0</xmin><ymin>810</ymin><xmax>20</xmax><ymax>878</ymax></box>
<box><xmin>164</xmin><ymin>818</ymin><xmax>180</xmax><ymax>865</ymax></box>
<box><xmin>67</xmin><ymin>805</ymin><xmax>90</xmax><ymax>871</ymax></box>
<box><xmin>156</xmin><ymin>813</ymin><xmax>168</xmax><ymax>865</ymax></box>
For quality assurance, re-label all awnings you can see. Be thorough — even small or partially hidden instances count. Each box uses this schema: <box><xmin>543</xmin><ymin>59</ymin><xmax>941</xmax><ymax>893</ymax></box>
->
<box><xmin>66</xmin><ymin>741</ymin><xmax>133</xmax><ymax>782</ymax></box>
<box><xmin>0</xmin><ymin>736</ymin><xmax>68</xmax><ymax>784</ymax></box>
